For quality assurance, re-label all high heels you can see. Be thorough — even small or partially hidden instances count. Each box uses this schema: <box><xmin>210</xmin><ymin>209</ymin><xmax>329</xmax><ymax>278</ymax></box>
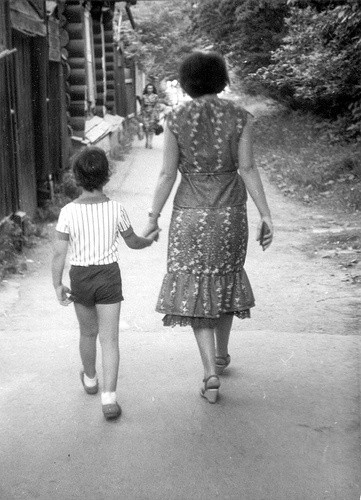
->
<box><xmin>201</xmin><ymin>373</ymin><xmax>220</xmax><ymax>403</ymax></box>
<box><xmin>214</xmin><ymin>352</ymin><xmax>232</xmax><ymax>375</ymax></box>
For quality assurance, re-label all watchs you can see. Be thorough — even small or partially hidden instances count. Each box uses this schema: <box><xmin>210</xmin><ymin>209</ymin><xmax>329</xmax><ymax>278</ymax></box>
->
<box><xmin>148</xmin><ymin>210</ymin><xmax>161</xmax><ymax>218</ymax></box>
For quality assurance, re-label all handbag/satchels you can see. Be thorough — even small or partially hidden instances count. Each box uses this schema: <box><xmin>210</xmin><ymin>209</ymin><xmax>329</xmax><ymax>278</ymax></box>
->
<box><xmin>155</xmin><ymin>127</ymin><xmax>164</xmax><ymax>135</ymax></box>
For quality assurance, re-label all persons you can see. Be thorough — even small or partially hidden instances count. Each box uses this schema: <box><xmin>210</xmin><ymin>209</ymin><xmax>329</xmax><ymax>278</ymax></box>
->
<box><xmin>140</xmin><ymin>83</ymin><xmax>160</xmax><ymax>149</ymax></box>
<box><xmin>52</xmin><ymin>145</ymin><xmax>163</xmax><ymax>420</ymax></box>
<box><xmin>140</xmin><ymin>49</ymin><xmax>274</xmax><ymax>403</ymax></box>
<box><xmin>163</xmin><ymin>78</ymin><xmax>187</xmax><ymax>122</ymax></box>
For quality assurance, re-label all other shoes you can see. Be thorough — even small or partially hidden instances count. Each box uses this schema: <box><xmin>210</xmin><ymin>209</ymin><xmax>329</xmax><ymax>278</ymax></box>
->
<box><xmin>148</xmin><ymin>144</ymin><xmax>152</xmax><ymax>148</ymax></box>
<box><xmin>79</xmin><ymin>368</ymin><xmax>99</xmax><ymax>395</ymax></box>
<box><xmin>146</xmin><ymin>142</ymin><xmax>149</xmax><ymax>148</ymax></box>
<box><xmin>101</xmin><ymin>402</ymin><xmax>121</xmax><ymax>418</ymax></box>
